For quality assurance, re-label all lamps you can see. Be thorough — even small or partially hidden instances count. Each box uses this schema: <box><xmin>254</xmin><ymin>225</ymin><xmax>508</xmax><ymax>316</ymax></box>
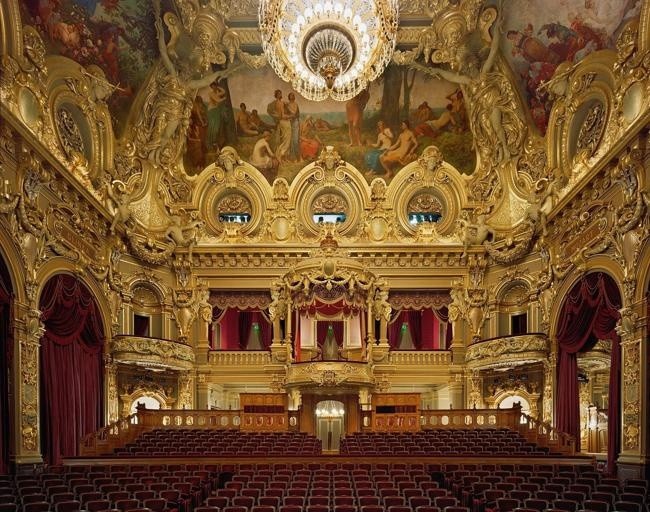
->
<box><xmin>257</xmin><ymin>0</ymin><xmax>399</xmax><ymax>103</ymax></box>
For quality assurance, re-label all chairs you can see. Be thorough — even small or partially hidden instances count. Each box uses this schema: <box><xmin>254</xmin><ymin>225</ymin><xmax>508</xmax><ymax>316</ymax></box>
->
<box><xmin>1</xmin><ymin>462</ymin><xmax>649</xmax><ymax>511</ymax></box>
<box><xmin>100</xmin><ymin>428</ymin><xmax>583</xmax><ymax>461</ymax></box>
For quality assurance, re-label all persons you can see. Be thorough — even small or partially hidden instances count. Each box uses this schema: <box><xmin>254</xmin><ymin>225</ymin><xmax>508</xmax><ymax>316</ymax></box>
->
<box><xmin>455</xmin><ymin>214</ymin><xmax>496</xmax><ymax>259</ymax></box>
<box><xmin>187</xmin><ymin>81</ymin><xmax>466</xmax><ymax>180</ymax></box>
<box><xmin>52</xmin><ymin>0</ymin><xmax>139</xmax><ymax>82</ymax></box>
<box><xmin>165</xmin><ymin>215</ymin><xmax>202</xmax><ymax>263</ymax></box>
<box><xmin>506</xmin><ymin>14</ymin><xmax>606</xmax><ymax>63</ymax></box>
